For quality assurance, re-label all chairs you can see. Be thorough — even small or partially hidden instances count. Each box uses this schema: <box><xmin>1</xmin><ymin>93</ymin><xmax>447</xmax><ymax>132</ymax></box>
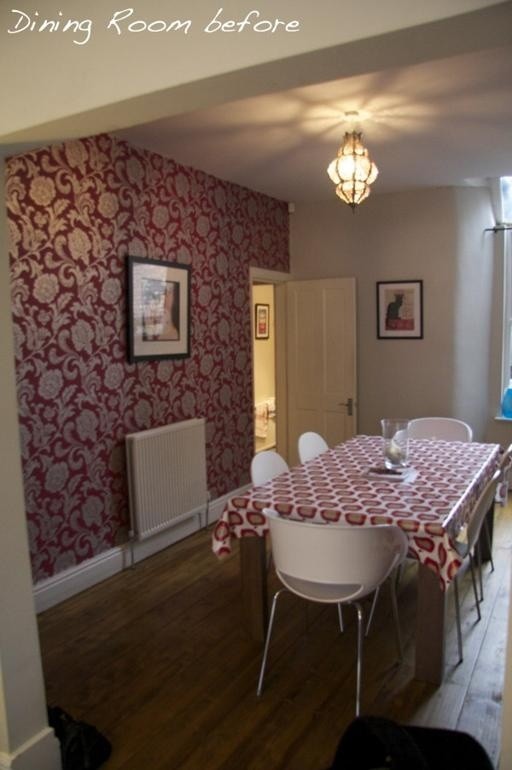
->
<box><xmin>250</xmin><ymin>451</ymin><xmax>292</xmax><ymax>491</ymax></box>
<box><xmin>256</xmin><ymin>508</ymin><xmax>410</xmax><ymax>719</ymax></box>
<box><xmin>406</xmin><ymin>416</ymin><xmax>475</xmax><ymax>447</ymax></box>
<box><xmin>298</xmin><ymin>432</ymin><xmax>330</xmax><ymax>468</ymax></box>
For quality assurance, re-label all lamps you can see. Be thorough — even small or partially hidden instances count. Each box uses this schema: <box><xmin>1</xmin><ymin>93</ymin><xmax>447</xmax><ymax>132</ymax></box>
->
<box><xmin>327</xmin><ymin>110</ymin><xmax>382</xmax><ymax>215</ymax></box>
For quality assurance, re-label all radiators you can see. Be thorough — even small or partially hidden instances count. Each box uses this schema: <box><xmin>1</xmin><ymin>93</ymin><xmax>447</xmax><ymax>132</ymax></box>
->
<box><xmin>123</xmin><ymin>417</ymin><xmax>209</xmax><ymax>542</ymax></box>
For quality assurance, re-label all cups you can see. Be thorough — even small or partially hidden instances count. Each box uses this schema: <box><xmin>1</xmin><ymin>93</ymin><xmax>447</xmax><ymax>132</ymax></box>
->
<box><xmin>381</xmin><ymin>417</ymin><xmax>411</xmax><ymax>470</ymax></box>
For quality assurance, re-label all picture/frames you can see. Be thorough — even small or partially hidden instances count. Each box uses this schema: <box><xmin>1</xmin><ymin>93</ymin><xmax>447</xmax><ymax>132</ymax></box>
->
<box><xmin>254</xmin><ymin>303</ymin><xmax>271</xmax><ymax>341</ymax></box>
<box><xmin>376</xmin><ymin>279</ymin><xmax>423</xmax><ymax>340</ymax></box>
<box><xmin>124</xmin><ymin>254</ymin><xmax>193</xmax><ymax>363</ymax></box>
